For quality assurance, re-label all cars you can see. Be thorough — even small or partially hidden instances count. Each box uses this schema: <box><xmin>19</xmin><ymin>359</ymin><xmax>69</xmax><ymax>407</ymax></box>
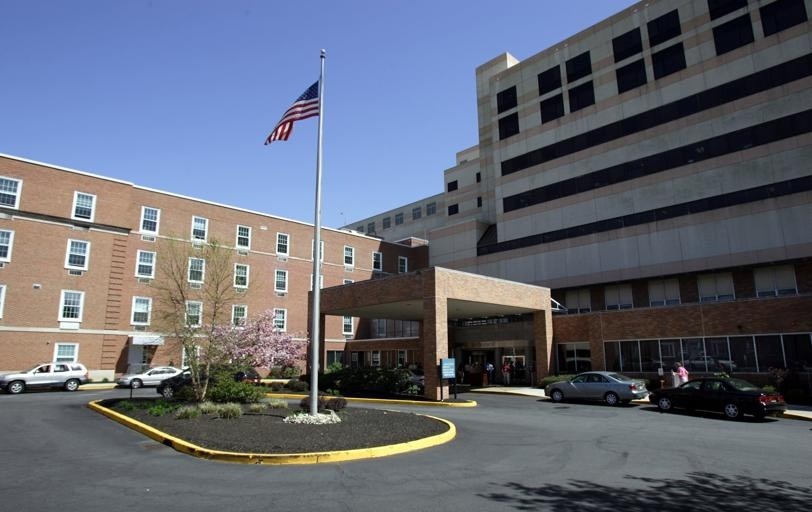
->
<box><xmin>393</xmin><ymin>366</ymin><xmax>425</xmax><ymax>387</ymax></box>
<box><xmin>117</xmin><ymin>364</ymin><xmax>191</xmax><ymax>388</ymax></box>
<box><xmin>156</xmin><ymin>362</ymin><xmax>264</xmax><ymax>398</ymax></box>
<box><xmin>545</xmin><ymin>368</ymin><xmax>650</xmax><ymax>407</ymax></box>
<box><xmin>621</xmin><ymin>355</ymin><xmax>666</xmax><ymax>369</ymax></box>
<box><xmin>647</xmin><ymin>375</ymin><xmax>788</xmax><ymax>422</ymax></box>
<box><xmin>680</xmin><ymin>355</ymin><xmax>737</xmax><ymax>371</ymax></box>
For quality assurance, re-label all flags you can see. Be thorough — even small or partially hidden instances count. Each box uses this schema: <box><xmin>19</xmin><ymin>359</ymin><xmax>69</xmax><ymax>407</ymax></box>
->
<box><xmin>264</xmin><ymin>78</ymin><xmax>319</xmax><ymax>144</ymax></box>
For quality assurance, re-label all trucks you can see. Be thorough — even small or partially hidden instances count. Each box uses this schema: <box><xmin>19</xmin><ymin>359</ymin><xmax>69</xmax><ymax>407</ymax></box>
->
<box><xmin>563</xmin><ymin>342</ymin><xmax>592</xmax><ymax>373</ymax></box>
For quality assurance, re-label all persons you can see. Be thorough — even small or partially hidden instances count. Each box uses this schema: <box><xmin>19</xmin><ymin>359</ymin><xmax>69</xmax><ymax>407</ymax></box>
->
<box><xmin>671</xmin><ymin>361</ymin><xmax>690</xmax><ymax>385</ymax></box>
<box><xmin>456</xmin><ymin>357</ymin><xmax>526</xmax><ymax>386</ymax></box>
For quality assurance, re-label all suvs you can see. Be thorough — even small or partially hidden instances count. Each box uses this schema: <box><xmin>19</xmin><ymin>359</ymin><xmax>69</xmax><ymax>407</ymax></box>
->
<box><xmin>0</xmin><ymin>359</ymin><xmax>89</xmax><ymax>395</ymax></box>
